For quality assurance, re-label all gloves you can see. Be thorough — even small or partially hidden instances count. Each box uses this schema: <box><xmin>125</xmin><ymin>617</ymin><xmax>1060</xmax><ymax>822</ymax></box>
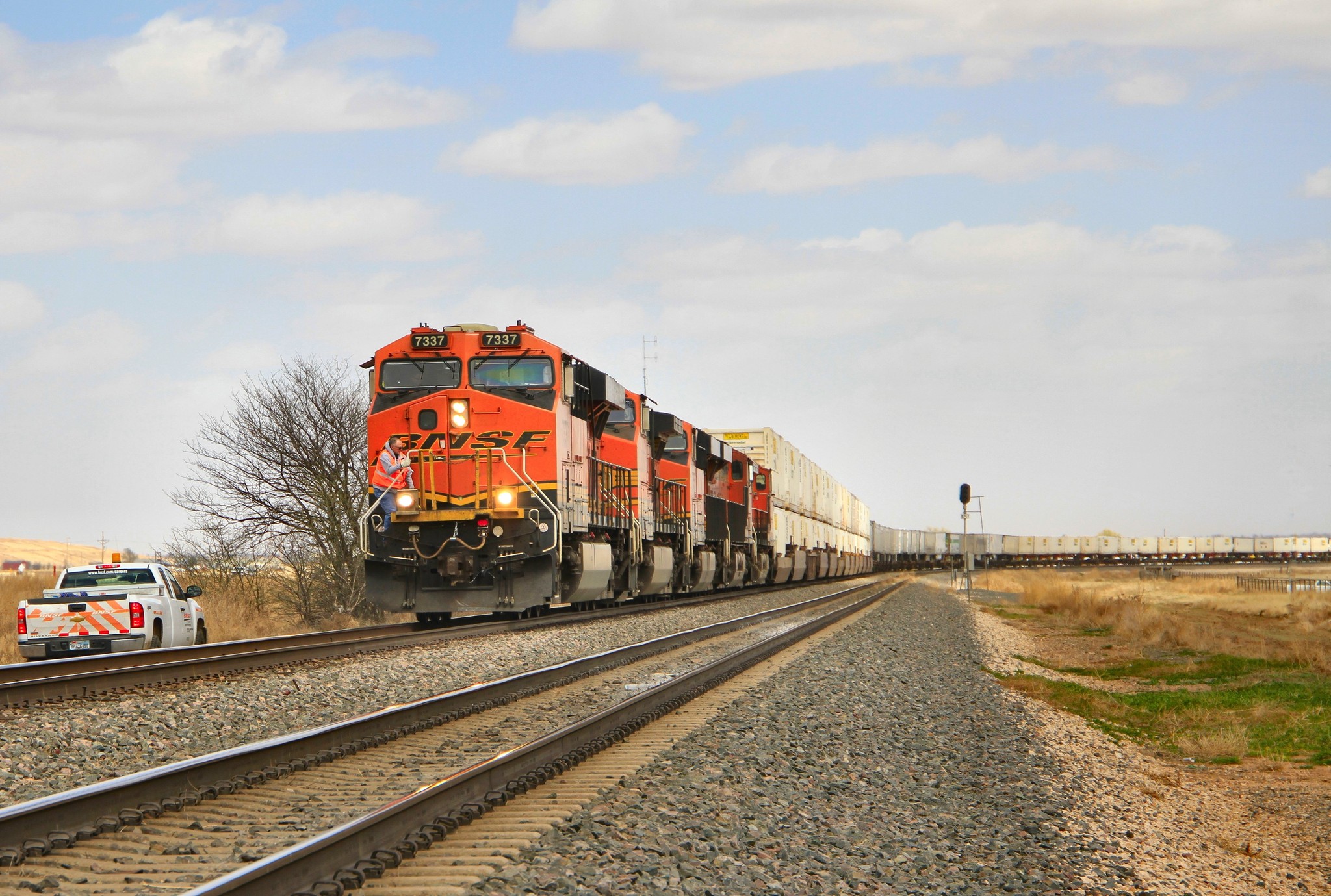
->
<box><xmin>401</xmin><ymin>459</ymin><xmax>412</xmax><ymax>468</ymax></box>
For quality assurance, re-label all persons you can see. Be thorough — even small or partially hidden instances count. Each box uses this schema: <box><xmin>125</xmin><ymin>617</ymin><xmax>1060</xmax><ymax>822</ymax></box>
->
<box><xmin>372</xmin><ymin>436</ymin><xmax>415</xmax><ymax>532</ymax></box>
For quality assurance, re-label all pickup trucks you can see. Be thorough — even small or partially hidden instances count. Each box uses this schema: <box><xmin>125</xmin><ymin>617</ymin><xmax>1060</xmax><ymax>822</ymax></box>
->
<box><xmin>17</xmin><ymin>553</ymin><xmax>208</xmax><ymax>659</ymax></box>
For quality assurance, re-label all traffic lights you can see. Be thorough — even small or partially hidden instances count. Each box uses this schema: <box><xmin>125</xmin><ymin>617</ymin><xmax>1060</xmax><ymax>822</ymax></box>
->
<box><xmin>960</xmin><ymin>483</ymin><xmax>971</xmax><ymax>504</ymax></box>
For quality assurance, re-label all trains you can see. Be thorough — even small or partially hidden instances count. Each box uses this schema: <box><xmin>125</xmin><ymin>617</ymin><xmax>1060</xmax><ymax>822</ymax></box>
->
<box><xmin>356</xmin><ymin>320</ymin><xmax>1330</xmax><ymax>623</ymax></box>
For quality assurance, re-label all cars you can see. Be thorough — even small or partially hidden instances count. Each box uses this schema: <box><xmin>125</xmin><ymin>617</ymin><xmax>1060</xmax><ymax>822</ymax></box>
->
<box><xmin>231</xmin><ymin>561</ymin><xmax>286</xmax><ymax>576</ymax></box>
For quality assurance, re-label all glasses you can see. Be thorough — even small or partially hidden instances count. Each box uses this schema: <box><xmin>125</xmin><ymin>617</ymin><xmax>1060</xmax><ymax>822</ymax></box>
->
<box><xmin>392</xmin><ymin>445</ymin><xmax>403</xmax><ymax>450</ymax></box>
